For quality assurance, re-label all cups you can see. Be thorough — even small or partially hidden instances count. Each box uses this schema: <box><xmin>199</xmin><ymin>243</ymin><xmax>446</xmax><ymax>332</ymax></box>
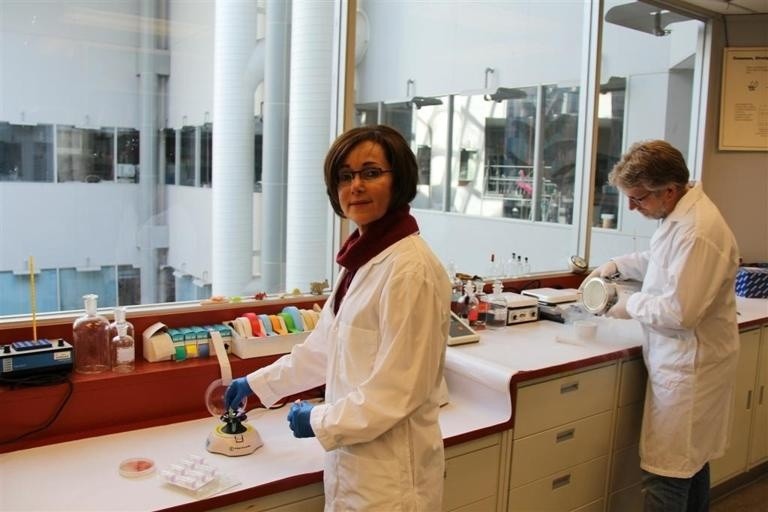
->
<box><xmin>573</xmin><ymin>319</ymin><xmax>598</xmax><ymax>339</ymax></box>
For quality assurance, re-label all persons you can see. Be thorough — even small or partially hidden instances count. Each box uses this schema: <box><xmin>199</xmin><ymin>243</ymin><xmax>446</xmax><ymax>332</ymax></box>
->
<box><xmin>224</xmin><ymin>125</ymin><xmax>452</xmax><ymax>512</ymax></box>
<box><xmin>580</xmin><ymin>139</ymin><xmax>740</xmax><ymax>512</ymax></box>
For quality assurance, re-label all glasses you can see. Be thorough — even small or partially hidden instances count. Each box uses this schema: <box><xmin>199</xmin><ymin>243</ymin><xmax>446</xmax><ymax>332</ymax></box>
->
<box><xmin>334</xmin><ymin>167</ymin><xmax>394</xmax><ymax>184</ymax></box>
<box><xmin>630</xmin><ymin>191</ymin><xmax>653</xmax><ymax>206</ymax></box>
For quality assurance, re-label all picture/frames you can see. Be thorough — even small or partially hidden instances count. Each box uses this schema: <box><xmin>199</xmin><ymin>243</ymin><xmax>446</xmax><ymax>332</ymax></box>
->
<box><xmin>716</xmin><ymin>48</ymin><xmax>768</xmax><ymax>152</ymax></box>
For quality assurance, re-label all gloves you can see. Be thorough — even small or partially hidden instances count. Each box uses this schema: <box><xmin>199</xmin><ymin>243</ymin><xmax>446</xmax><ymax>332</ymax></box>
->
<box><xmin>578</xmin><ymin>259</ymin><xmax>618</xmax><ymax>291</ymax></box>
<box><xmin>287</xmin><ymin>399</ymin><xmax>316</xmax><ymax>439</ymax></box>
<box><xmin>605</xmin><ymin>297</ymin><xmax>632</xmax><ymax>320</ymax></box>
<box><xmin>223</xmin><ymin>375</ymin><xmax>253</xmax><ymax>411</ymax></box>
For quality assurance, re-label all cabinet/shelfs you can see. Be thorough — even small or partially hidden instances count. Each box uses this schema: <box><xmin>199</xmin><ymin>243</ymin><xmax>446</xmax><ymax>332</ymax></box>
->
<box><xmin>486</xmin><ymin>166</ymin><xmax>556</xmax><ymax>220</ymax></box>
<box><xmin>158</xmin><ymin>472</ymin><xmax>324</xmax><ymax>512</ymax></box>
<box><xmin>607</xmin><ymin>347</ymin><xmax>648</xmax><ymax>512</ymax></box>
<box><xmin>443</xmin><ymin>423</ymin><xmax>502</xmax><ymax>512</ymax></box>
<box><xmin>710</xmin><ymin>317</ymin><xmax>768</xmax><ymax>505</ymax></box>
<box><xmin>508</xmin><ymin>349</ymin><xmax>619</xmax><ymax>512</ymax></box>
